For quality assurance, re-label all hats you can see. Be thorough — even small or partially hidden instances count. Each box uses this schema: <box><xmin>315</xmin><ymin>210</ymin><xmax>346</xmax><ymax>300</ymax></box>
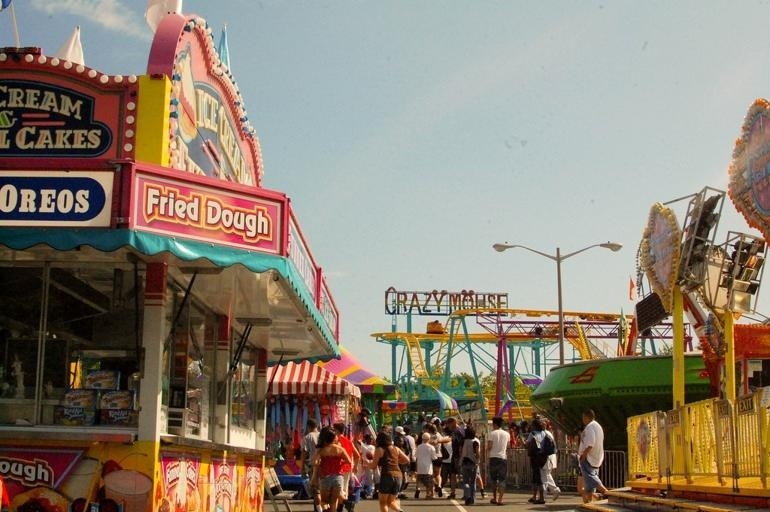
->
<box><xmin>395</xmin><ymin>426</ymin><xmax>406</xmax><ymax>435</ymax></box>
<box><xmin>431</xmin><ymin>417</ymin><xmax>441</xmax><ymax>424</ymax></box>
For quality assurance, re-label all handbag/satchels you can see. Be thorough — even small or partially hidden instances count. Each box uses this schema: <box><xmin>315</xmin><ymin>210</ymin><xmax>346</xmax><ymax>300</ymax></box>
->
<box><xmin>527</xmin><ymin>438</ymin><xmax>538</xmax><ymax>457</ymax></box>
<box><xmin>542</xmin><ymin>434</ymin><xmax>554</xmax><ymax>455</ymax></box>
<box><xmin>441</xmin><ymin>443</ymin><xmax>449</xmax><ymax>459</ymax></box>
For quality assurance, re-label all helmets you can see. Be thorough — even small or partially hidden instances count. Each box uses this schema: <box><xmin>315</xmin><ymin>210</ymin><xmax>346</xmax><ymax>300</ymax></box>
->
<box><xmin>361</xmin><ymin>408</ymin><xmax>372</xmax><ymax>415</ymax></box>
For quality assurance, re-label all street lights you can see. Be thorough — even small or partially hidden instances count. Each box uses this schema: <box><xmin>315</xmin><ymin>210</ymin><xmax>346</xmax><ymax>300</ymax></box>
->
<box><xmin>493</xmin><ymin>240</ymin><xmax>623</xmax><ymax>366</ymax></box>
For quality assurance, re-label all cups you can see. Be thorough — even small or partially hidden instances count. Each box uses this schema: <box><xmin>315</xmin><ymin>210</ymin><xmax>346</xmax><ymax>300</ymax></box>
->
<box><xmin>60</xmin><ymin>456</ymin><xmax>105</xmax><ymax>511</ymax></box>
<box><xmin>211</xmin><ymin>453</ymin><xmax>237</xmax><ymax>512</ymax></box>
<box><xmin>103</xmin><ymin>471</ymin><xmax>153</xmax><ymax>512</ymax></box>
<box><xmin>160</xmin><ymin>452</ymin><xmax>200</xmax><ymax>512</ymax></box>
<box><xmin>245</xmin><ymin>460</ymin><xmax>263</xmax><ymax>512</ymax></box>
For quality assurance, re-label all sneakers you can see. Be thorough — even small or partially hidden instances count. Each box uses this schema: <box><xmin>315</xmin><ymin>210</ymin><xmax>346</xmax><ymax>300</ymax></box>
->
<box><xmin>415</xmin><ymin>483</ymin><xmax>503</xmax><ymax>505</ymax></box>
<box><xmin>360</xmin><ymin>490</ymin><xmax>408</xmax><ymax>499</ymax></box>
<box><xmin>528</xmin><ymin>489</ymin><xmax>561</xmax><ymax>504</ymax></box>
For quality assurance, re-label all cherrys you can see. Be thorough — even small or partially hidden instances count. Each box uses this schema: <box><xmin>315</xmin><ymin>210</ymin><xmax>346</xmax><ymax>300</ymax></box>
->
<box><xmin>71</xmin><ymin>497</ymin><xmax>90</xmax><ymax>512</ymax></box>
<box><xmin>99</xmin><ymin>498</ymin><xmax>119</xmax><ymax>512</ymax></box>
<box><xmin>102</xmin><ymin>452</ymin><xmax>148</xmax><ymax>477</ymax></box>
